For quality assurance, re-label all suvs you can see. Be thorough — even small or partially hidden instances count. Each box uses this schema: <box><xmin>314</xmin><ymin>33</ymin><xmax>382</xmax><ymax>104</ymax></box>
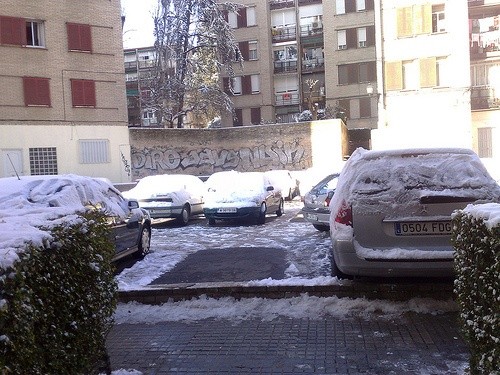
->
<box><xmin>330</xmin><ymin>148</ymin><xmax>500</xmax><ymax>278</ymax></box>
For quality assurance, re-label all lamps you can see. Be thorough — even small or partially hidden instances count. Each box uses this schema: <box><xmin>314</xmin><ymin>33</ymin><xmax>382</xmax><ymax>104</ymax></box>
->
<box><xmin>366</xmin><ymin>83</ymin><xmax>380</xmax><ymax>97</ymax></box>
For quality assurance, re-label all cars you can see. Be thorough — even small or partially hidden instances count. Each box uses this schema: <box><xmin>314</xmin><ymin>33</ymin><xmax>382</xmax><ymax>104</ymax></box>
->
<box><xmin>203</xmin><ymin>169</ymin><xmax>299</xmax><ymax>223</ymax></box>
<box><xmin>119</xmin><ymin>174</ymin><xmax>203</xmax><ymax>225</ymax></box>
<box><xmin>0</xmin><ymin>174</ymin><xmax>151</xmax><ymax>302</ymax></box>
<box><xmin>302</xmin><ymin>172</ymin><xmax>340</xmax><ymax>230</ymax></box>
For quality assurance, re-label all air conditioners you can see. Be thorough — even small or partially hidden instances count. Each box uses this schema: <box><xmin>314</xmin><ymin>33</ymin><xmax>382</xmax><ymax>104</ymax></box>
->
<box><xmin>311</xmin><ymin>21</ymin><xmax>322</xmax><ymax>29</ymax></box>
